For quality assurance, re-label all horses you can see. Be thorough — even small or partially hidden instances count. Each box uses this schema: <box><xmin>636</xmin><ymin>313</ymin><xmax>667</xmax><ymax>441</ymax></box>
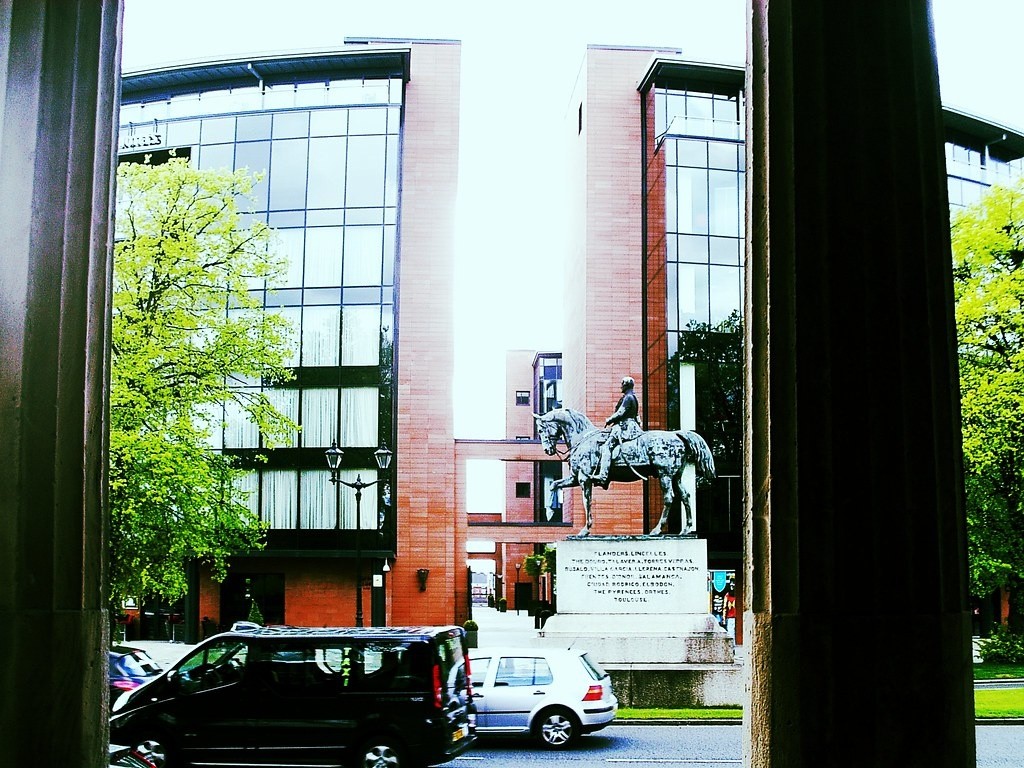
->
<box><xmin>530</xmin><ymin>408</ymin><xmax>718</xmax><ymax>537</ymax></box>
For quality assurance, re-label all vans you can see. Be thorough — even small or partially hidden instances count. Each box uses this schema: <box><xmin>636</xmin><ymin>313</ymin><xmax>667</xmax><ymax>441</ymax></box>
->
<box><xmin>108</xmin><ymin>624</ymin><xmax>480</xmax><ymax>768</ymax></box>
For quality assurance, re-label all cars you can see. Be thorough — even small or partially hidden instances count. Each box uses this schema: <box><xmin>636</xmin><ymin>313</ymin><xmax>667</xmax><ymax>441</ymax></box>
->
<box><xmin>465</xmin><ymin>645</ymin><xmax>620</xmax><ymax>748</ymax></box>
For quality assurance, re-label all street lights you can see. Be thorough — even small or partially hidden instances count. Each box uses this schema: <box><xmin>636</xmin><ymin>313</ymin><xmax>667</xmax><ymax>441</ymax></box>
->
<box><xmin>326</xmin><ymin>438</ymin><xmax>393</xmax><ymax>629</ymax></box>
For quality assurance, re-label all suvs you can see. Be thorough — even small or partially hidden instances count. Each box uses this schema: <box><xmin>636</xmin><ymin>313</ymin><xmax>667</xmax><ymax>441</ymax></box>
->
<box><xmin>109</xmin><ymin>641</ymin><xmax>163</xmax><ymax>710</ymax></box>
<box><xmin>108</xmin><ymin>744</ymin><xmax>158</xmax><ymax>768</ymax></box>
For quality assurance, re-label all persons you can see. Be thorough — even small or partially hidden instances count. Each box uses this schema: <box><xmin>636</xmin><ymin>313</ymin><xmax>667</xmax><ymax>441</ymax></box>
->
<box><xmin>591</xmin><ymin>377</ymin><xmax>641</xmax><ymax>491</ymax></box>
